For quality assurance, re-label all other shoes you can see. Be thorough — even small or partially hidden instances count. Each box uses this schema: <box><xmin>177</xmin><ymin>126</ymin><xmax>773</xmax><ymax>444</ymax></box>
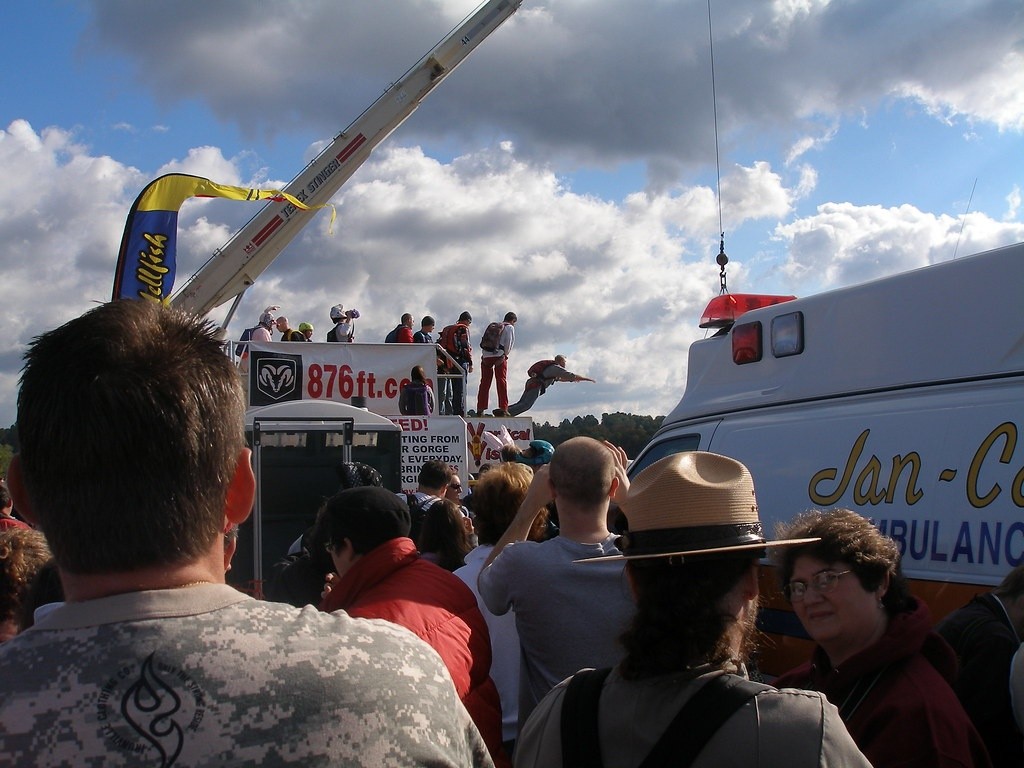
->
<box><xmin>492</xmin><ymin>409</ymin><xmax>504</xmax><ymax>416</ymax></box>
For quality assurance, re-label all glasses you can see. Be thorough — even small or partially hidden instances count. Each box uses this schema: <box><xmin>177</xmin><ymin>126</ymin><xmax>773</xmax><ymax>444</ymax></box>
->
<box><xmin>786</xmin><ymin>571</ymin><xmax>854</xmax><ymax>595</ymax></box>
<box><xmin>450</xmin><ymin>484</ymin><xmax>462</xmax><ymax>490</ymax></box>
<box><xmin>326</xmin><ymin>542</ymin><xmax>336</xmax><ymax>553</ymax></box>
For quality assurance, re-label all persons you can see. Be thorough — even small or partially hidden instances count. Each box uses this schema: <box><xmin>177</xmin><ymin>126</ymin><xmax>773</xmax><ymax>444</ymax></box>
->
<box><xmin>276</xmin><ymin>317</ymin><xmax>306</xmax><ymax>341</ymax></box>
<box><xmin>330</xmin><ymin>304</ymin><xmax>355</xmax><ymax>342</ymax></box>
<box><xmin>0</xmin><ymin>478</ymin><xmax>65</xmax><ymax>644</ymax></box>
<box><xmin>0</xmin><ymin>301</ymin><xmax>494</xmax><ymax>768</ymax></box>
<box><xmin>476</xmin><ymin>436</ymin><xmax>633</xmax><ymax>739</ymax></box>
<box><xmin>493</xmin><ymin>355</ymin><xmax>595</xmax><ymax>416</ymax></box>
<box><xmin>396</xmin><ymin>313</ymin><xmax>414</xmax><ymax>342</ymax></box>
<box><xmin>435</xmin><ymin>310</ymin><xmax>473</xmax><ymax>417</ymax></box>
<box><xmin>252</xmin><ymin>305</ymin><xmax>280</xmax><ymax>341</ymax></box>
<box><xmin>935</xmin><ymin>564</ymin><xmax>1024</xmax><ymax>768</ymax></box>
<box><xmin>399</xmin><ymin>366</ymin><xmax>435</xmax><ymax>416</ymax></box>
<box><xmin>314</xmin><ymin>486</ymin><xmax>512</xmax><ymax>768</ymax></box>
<box><xmin>767</xmin><ymin>509</ymin><xmax>992</xmax><ymax>768</ymax></box>
<box><xmin>299</xmin><ymin>322</ymin><xmax>314</xmax><ymax>342</ymax></box>
<box><xmin>414</xmin><ymin>316</ymin><xmax>444</xmax><ymax>365</ymax></box>
<box><xmin>477</xmin><ymin>312</ymin><xmax>514</xmax><ymax>418</ymax></box>
<box><xmin>515</xmin><ymin>451</ymin><xmax>874</xmax><ymax>768</ymax></box>
<box><xmin>269</xmin><ymin>440</ymin><xmax>559</xmax><ymax>754</ymax></box>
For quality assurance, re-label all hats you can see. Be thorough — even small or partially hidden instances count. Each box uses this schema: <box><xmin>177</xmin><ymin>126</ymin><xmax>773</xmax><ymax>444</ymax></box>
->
<box><xmin>459</xmin><ymin>312</ymin><xmax>471</xmax><ymax>321</ymax></box>
<box><xmin>422</xmin><ymin>316</ymin><xmax>434</xmax><ymax>327</ymax></box>
<box><xmin>571</xmin><ymin>452</ymin><xmax>822</xmax><ymax>564</ymax></box>
<box><xmin>331</xmin><ymin>462</ymin><xmax>384</xmax><ymax>493</ymax></box>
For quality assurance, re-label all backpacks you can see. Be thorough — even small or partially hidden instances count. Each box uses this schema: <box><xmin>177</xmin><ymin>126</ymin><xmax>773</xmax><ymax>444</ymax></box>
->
<box><xmin>236</xmin><ymin>327</ymin><xmax>264</xmax><ymax>357</ymax></box>
<box><xmin>385</xmin><ymin>324</ymin><xmax>407</xmax><ymax>343</ymax></box>
<box><xmin>527</xmin><ymin>360</ymin><xmax>559</xmax><ymax>380</ymax></box>
<box><xmin>480</xmin><ymin>323</ymin><xmax>512</xmax><ymax>354</ymax></box>
<box><xmin>327</xmin><ymin>323</ymin><xmax>343</xmax><ymax>342</ymax></box>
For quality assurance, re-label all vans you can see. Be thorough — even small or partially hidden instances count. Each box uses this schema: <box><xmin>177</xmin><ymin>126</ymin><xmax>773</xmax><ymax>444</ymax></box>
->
<box><xmin>622</xmin><ymin>241</ymin><xmax>1024</xmax><ymax>629</ymax></box>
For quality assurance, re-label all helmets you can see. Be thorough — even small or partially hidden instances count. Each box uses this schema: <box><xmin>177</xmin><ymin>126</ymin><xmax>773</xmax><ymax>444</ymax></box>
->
<box><xmin>331</xmin><ymin>304</ymin><xmax>347</xmax><ymax>319</ymax></box>
<box><xmin>259</xmin><ymin>313</ymin><xmax>274</xmax><ymax>323</ymax></box>
<box><xmin>516</xmin><ymin>442</ymin><xmax>555</xmax><ymax>465</ymax></box>
<box><xmin>299</xmin><ymin>322</ymin><xmax>314</xmax><ymax>332</ymax></box>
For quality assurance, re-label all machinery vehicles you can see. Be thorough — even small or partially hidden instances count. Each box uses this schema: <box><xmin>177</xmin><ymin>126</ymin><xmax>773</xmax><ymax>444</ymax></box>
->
<box><xmin>169</xmin><ymin>0</ymin><xmax>540</xmax><ymax>607</ymax></box>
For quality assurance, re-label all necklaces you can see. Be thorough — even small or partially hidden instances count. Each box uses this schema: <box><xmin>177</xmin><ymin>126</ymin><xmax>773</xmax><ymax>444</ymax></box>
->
<box><xmin>180</xmin><ymin>581</ymin><xmax>209</xmax><ymax>587</ymax></box>
